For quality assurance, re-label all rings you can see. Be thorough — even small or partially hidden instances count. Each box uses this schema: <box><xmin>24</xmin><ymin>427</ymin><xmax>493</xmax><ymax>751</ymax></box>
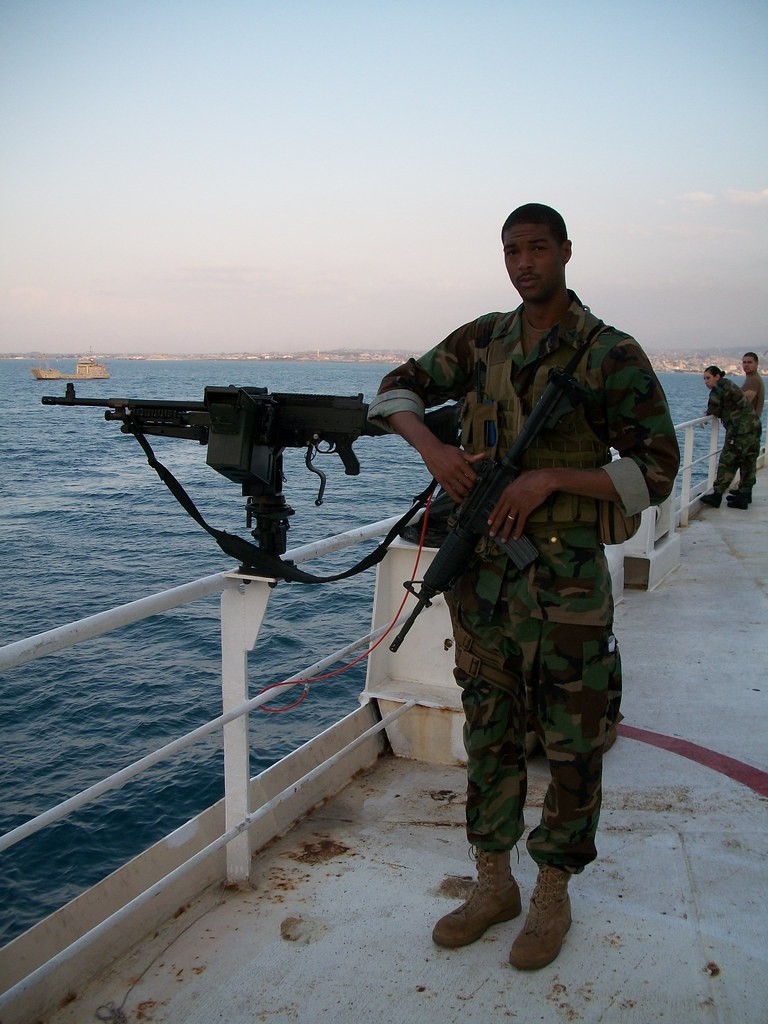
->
<box><xmin>508</xmin><ymin>515</ymin><xmax>515</xmax><ymax>519</ymax></box>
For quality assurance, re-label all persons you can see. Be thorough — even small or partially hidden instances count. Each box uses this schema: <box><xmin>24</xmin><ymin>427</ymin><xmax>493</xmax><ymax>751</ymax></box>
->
<box><xmin>726</xmin><ymin>352</ymin><xmax>765</xmax><ymax>504</ymax></box>
<box><xmin>368</xmin><ymin>202</ymin><xmax>680</xmax><ymax>969</ymax></box>
<box><xmin>697</xmin><ymin>366</ymin><xmax>762</xmax><ymax>510</ymax></box>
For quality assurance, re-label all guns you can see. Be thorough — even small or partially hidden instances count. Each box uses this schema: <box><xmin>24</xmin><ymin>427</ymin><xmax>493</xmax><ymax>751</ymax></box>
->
<box><xmin>387</xmin><ymin>366</ymin><xmax>591</xmax><ymax>654</ymax></box>
<box><xmin>41</xmin><ymin>382</ymin><xmax>461</xmax><ymax>506</ymax></box>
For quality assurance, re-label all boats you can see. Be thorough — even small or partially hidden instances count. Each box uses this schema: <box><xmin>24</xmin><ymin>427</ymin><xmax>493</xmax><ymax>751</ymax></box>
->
<box><xmin>29</xmin><ymin>356</ymin><xmax>110</xmax><ymax>379</ymax></box>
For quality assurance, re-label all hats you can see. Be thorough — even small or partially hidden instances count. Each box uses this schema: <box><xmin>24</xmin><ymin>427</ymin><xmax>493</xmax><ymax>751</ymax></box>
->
<box><xmin>399</xmin><ymin>488</ymin><xmax>464</xmax><ymax>548</ymax></box>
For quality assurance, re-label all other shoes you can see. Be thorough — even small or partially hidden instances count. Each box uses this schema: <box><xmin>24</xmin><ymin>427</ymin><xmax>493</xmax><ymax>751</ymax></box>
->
<box><xmin>726</xmin><ymin>489</ymin><xmax>752</xmax><ymax>503</ymax></box>
<box><xmin>697</xmin><ymin>492</ymin><xmax>722</xmax><ymax>508</ymax></box>
<box><xmin>727</xmin><ymin>492</ymin><xmax>748</xmax><ymax>509</ymax></box>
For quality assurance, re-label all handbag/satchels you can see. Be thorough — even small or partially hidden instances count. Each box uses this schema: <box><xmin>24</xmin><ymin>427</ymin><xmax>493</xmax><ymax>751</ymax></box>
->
<box><xmin>599</xmin><ymin>499</ymin><xmax>641</xmax><ymax>545</ymax></box>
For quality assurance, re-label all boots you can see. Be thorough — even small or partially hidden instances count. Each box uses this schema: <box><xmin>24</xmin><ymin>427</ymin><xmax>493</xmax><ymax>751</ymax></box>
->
<box><xmin>432</xmin><ymin>842</ymin><xmax>521</xmax><ymax>948</ymax></box>
<box><xmin>509</xmin><ymin>864</ymin><xmax>571</xmax><ymax>970</ymax></box>
